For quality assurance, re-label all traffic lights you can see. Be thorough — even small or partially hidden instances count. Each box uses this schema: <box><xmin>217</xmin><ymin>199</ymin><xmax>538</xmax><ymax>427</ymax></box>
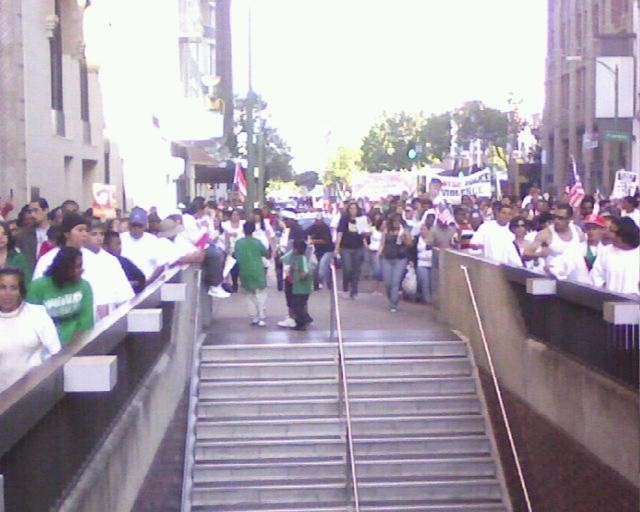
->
<box><xmin>407</xmin><ymin>139</ymin><xmax>417</xmax><ymax>159</ymax></box>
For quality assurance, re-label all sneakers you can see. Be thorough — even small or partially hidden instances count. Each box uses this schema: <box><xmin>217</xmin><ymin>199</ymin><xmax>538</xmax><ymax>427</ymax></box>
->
<box><xmin>259</xmin><ymin>320</ymin><xmax>265</xmax><ymax>326</ymax></box>
<box><xmin>208</xmin><ymin>286</ymin><xmax>231</xmax><ymax>298</ymax></box>
<box><xmin>277</xmin><ymin>314</ymin><xmax>313</xmax><ymax>330</ymax></box>
<box><xmin>252</xmin><ymin>319</ymin><xmax>257</xmax><ymax>324</ymax></box>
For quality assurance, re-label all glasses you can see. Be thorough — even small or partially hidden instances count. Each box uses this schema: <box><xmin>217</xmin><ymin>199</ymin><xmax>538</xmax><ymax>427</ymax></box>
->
<box><xmin>516</xmin><ymin>224</ymin><xmax>525</xmax><ymax>227</ymax></box>
<box><xmin>552</xmin><ymin>214</ymin><xmax>565</xmax><ymax>220</ymax></box>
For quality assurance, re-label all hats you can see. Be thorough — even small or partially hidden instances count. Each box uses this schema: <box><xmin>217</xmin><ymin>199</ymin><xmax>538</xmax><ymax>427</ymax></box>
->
<box><xmin>129</xmin><ymin>209</ymin><xmax>144</xmax><ymax>226</ymax></box>
<box><xmin>157</xmin><ymin>219</ymin><xmax>184</xmax><ymax>238</ymax></box>
<box><xmin>582</xmin><ymin>214</ymin><xmax>605</xmax><ymax>227</ymax></box>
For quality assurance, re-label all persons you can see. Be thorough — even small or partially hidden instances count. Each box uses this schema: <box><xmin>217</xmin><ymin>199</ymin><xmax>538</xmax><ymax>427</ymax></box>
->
<box><xmin>220</xmin><ymin>195</ymin><xmax>449</xmax><ymax>330</ymax></box>
<box><xmin>434</xmin><ymin>192</ymin><xmax>640</xmax><ymax>300</ymax></box>
<box><xmin>2</xmin><ymin>196</ymin><xmax>236</xmax><ymax>391</ymax></box>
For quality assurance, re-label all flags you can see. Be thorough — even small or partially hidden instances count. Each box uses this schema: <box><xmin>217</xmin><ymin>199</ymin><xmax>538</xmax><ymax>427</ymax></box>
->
<box><xmin>563</xmin><ymin>161</ymin><xmax>585</xmax><ymax>209</ymax></box>
<box><xmin>234</xmin><ymin>165</ymin><xmax>249</xmax><ymax>196</ymax></box>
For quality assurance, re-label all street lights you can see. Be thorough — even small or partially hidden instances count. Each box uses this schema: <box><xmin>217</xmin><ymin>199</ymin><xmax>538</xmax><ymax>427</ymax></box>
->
<box><xmin>564</xmin><ymin>56</ymin><xmax>619</xmax><ymax>194</ymax></box>
